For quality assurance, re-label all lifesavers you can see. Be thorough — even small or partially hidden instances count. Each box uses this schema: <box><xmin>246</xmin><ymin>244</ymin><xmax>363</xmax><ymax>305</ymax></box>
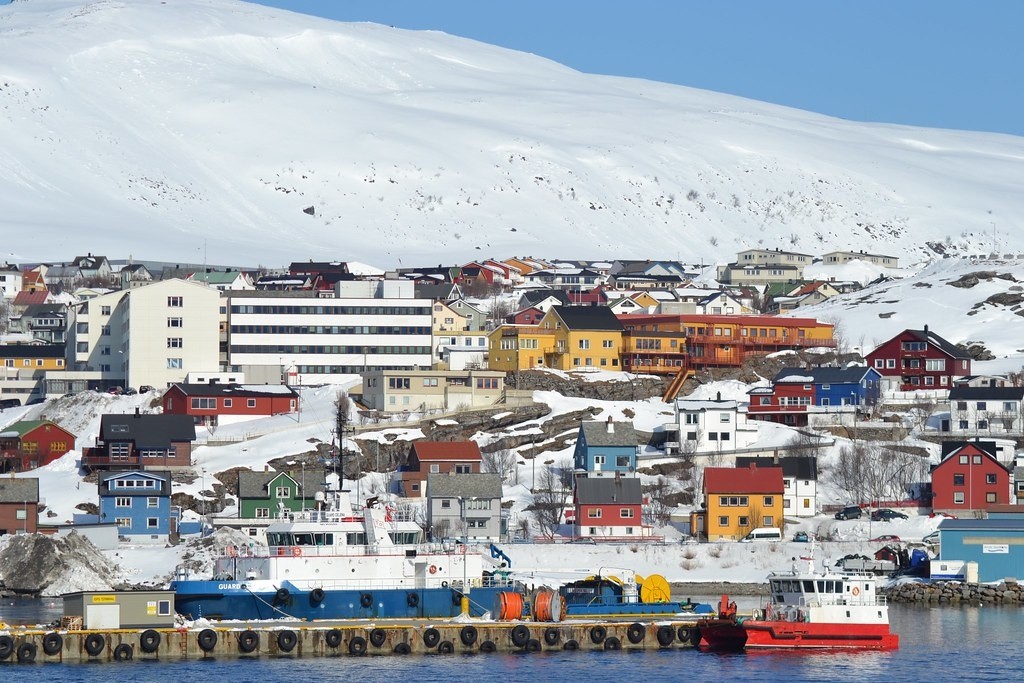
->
<box><xmin>349</xmin><ymin>637</ymin><xmax>367</xmax><ymax>653</ymax></box>
<box><xmin>430</xmin><ymin>565</ymin><xmax>437</xmax><ymax>573</ymax></box>
<box><xmin>239</xmin><ymin>630</ymin><xmax>259</xmax><ymax>652</ymax></box>
<box><xmin>293</xmin><ymin>547</ymin><xmax>302</xmax><ymax>556</ymax></box>
<box><xmin>326</xmin><ymin>629</ymin><xmax>342</xmax><ymax>648</ymax></box>
<box><xmin>423</xmin><ymin>629</ymin><xmax>440</xmax><ymax>647</ymax></box>
<box><xmin>278</xmin><ymin>630</ymin><xmax>297</xmax><ymax>653</ymax></box>
<box><xmin>198</xmin><ymin>629</ymin><xmax>217</xmax><ymax>650</ymax></box>
<box><xmin>114</xmin><ymin>643</ymin><xmax>132</xmax><ymax>660</ymax></box>
<box><xmin>277</xmin><ymin>588</ymin><xmax>466</xmax><ymax>605</ymax></box>
<box><xmin>0</xmin><ymin>636</ymin><xmax>14</xmax><ymax>659</ymax></box>
<box><xmin>394</xmin><ymin>643</ymin><xmax>412</xmax><ymax>653</ymax></box>
<box><xmin>852</xmin><ymin>587</ymin><xmax>859</xmax><ymax>595</ymax></box>
<box><xmin>140</xmin><ymin>630</ymin><xmax>161</xmax><ymax>653</ymax></box>
<box><xmin>85</xmin><ymin>633</ymin><xmax>105</xmax><ymax>655</ymax></box>
<box><xmin>370</xmin><ymin>628</ymin><xmax>387</xmax><ymax>646</ymax></box>
<box><xmin>393</xmin><ymin>626</ymin><xmax>693</xmax><ymax>653</ymax></box>
<box><xmin>17</xmin><ymin>643</ymin><xmax>36</xmax><ymax>662</ymax></box>
<box><xmin>43</xmin><ymin>633</ymin><xmax>63</xmax><ymax>655</ymax></box>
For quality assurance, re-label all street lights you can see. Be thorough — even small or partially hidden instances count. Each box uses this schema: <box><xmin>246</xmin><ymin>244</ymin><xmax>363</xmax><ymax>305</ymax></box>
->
<box><xmin>200</xmin><ymin>467</ymin><xmax>208</xmax><ymax>537</ymax></box>
<box><xmin>851</xmin><ymin>392</ymin><xmax>858</xmax><ymax>444</ymax></box>
<box><xmin>301</xmin><ymin>462</ymin><xmax>305</xmax><ymax>520</ymax></box>
<box><xmin>456</xmin><ymin>495</ymin><xmax>478</xmax><ymax>587</ymax></box>
<box><xmin>569</xmin><ymin>466</ymin><xmax>587</xmax><ymax>540</ymax></box>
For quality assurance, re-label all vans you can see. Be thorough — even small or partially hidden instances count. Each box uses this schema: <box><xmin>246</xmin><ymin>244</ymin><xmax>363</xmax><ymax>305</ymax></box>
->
<box><xmin>0</xmin><ymin>398</ymin><xmax>21</xmax><ymax>409</ymax></box>
<box><xmin>738</xmin><ymin>527</ymin><xmax>782</xmax><ymax>543</ymax></box>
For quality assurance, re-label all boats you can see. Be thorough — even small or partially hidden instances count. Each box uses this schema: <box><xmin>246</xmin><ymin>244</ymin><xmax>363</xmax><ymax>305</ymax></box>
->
<box><xmin>167</xmin><ymin>486</ymin><xmax>538</xmax><ymax>619</ymax></box>
<box><xmin>697</xmin><ymin>532</ymin><xmax>903</xmax><ymax>652</ymax></box>
<box><xmin>558</xmin><ymin>566</ymin><xmax>716</xmax><ymax>616</ymax></box>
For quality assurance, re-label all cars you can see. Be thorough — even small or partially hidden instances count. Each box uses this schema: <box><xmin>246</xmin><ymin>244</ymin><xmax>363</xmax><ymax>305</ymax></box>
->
<box><xmin>94</xmin><ymin>385</ymin><xmax>156</xmax><ymax>396</ymax></box>
<box><xmin>564</xmin><ymin>536</ymin><xmax>596</xmax><ymax>545</ymax></box>
<box><xmin>929</xmin><ymin>511</ymin><xmax>958</xmax><ymax>519</ymax></box>
<box><xmin>871</xmin><ymin>508</ymin><xmax>909</xmax><ymax>521</ymax></box>
<box><xmin>26</xmin><ymin>397</ymin><xmax>47</xmax><ymax>405</ymax></box>
<box><xmin>792</xmin><ymin>531</ymin><xmax>809</xmax><ymax>542</ymax></box>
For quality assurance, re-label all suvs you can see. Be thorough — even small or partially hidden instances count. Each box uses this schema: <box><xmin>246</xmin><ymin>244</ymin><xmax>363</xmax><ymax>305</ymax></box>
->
<box><xmin>835</xmin><ymin>505</ymin><xmax>862</xmax><ymax>520</ymax></box>
